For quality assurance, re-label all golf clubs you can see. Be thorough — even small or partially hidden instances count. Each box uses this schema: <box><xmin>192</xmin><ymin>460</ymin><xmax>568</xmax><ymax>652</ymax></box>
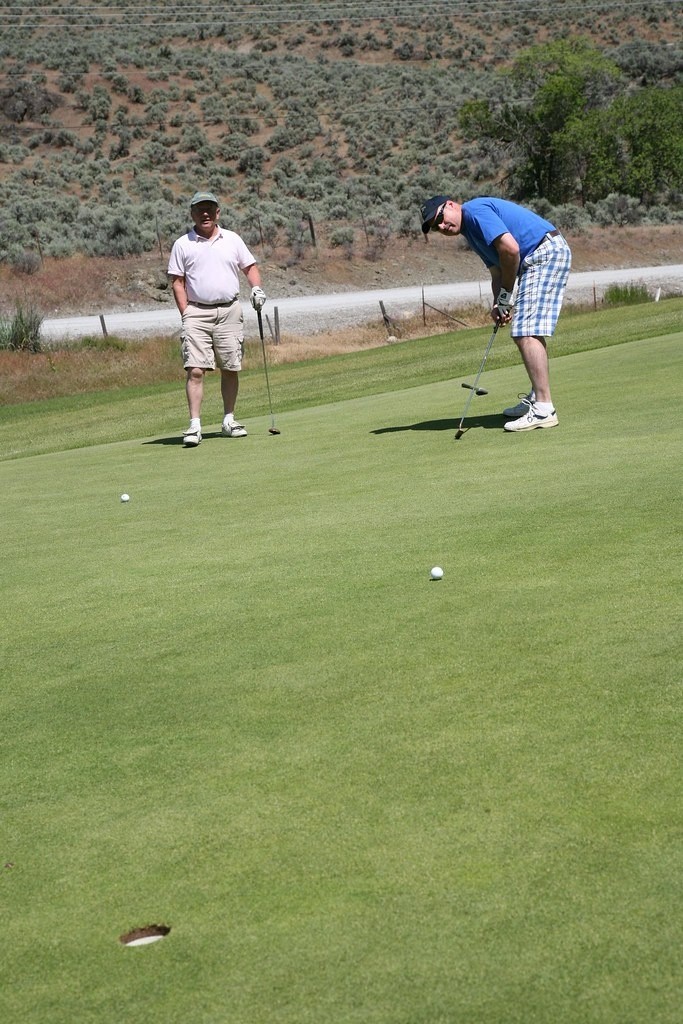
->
<box><xmin>252</xmin><ymin>298</ymin><xmax>281</xmax><ymax>435</ymax></box>
<box><xmin>455</xmin><ymin>318</ymin><xmax>501</xmax><ymax>440</ymax></box>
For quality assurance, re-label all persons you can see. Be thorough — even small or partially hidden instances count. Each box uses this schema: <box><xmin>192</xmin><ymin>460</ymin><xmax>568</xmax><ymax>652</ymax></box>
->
<box><xmin>421</xmin><ymin>195</ymin><xmax>573</xmax><ymax>432</ymax></box>
<box><xmin>167</xmin><ymin>191</ymin><xmax>267</xmax><ymax>447</ymax></box>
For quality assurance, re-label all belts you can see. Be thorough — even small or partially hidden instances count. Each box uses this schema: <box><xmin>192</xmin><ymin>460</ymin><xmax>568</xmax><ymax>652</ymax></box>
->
<box><xmin>534</xmin><ymin>229</ymin><xmax>560</xmax><ymax>251</ymax></box>
<box><xmin>188</xmin><ymin>297</ymin><xmax>237</xmax><ymax>310</ymax></box>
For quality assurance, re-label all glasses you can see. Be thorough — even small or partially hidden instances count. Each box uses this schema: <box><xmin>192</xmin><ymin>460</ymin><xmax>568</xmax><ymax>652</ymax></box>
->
<box><xmin>431</xmin><ymin>203</ymin><xmax>446</xmax><ymax>232</ymax></box>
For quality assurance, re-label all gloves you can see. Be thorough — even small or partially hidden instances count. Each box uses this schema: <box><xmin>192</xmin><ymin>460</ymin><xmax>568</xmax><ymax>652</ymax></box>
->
<box><xmin>250</xmin><ymin>286</ymin><xmax>266</xmax><ymax>312</ymax></box>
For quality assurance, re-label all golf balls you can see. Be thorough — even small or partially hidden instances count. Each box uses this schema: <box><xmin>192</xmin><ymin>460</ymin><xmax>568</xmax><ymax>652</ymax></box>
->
<box><xmin>121</xmin><ymin>494</ymin><xmax>129</xmax><ymax>502</ymax></box>
<box><xmin>429</xmin><ymin>566</ymin><xmax>443</xmax><ymax>581</ymax></box>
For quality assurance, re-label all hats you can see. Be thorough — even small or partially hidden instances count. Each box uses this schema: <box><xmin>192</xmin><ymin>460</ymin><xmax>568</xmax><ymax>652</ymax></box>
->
<box><xmin>420</xmin><ymin>195</ymin><xmax>452</xmax><ymax>234</ymax></box>
<box><xmin>190</xmin><ymin>191</ymin><xmax>219</xmax><ymax>208</ymax></box>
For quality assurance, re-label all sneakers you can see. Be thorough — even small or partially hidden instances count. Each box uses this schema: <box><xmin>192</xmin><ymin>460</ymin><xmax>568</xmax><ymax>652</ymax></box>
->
<box><xmin>183</xmin><ymin>426</ymin><xmax>202</xmax><ymax>445</ymax></box>
<box><xmin>503</xmin><ymin>393</ymin><xmax>536</xmax><ymax>416</ymax></box>
<box><xmin>504</xmin><ymin>398</ymin><xmax>558</xmax><ymax>431</ymax></box>
<box><xmin>221</xmin><ymin>420</ymin><xmax>247</xmax><ymax>437</ymax></box>
